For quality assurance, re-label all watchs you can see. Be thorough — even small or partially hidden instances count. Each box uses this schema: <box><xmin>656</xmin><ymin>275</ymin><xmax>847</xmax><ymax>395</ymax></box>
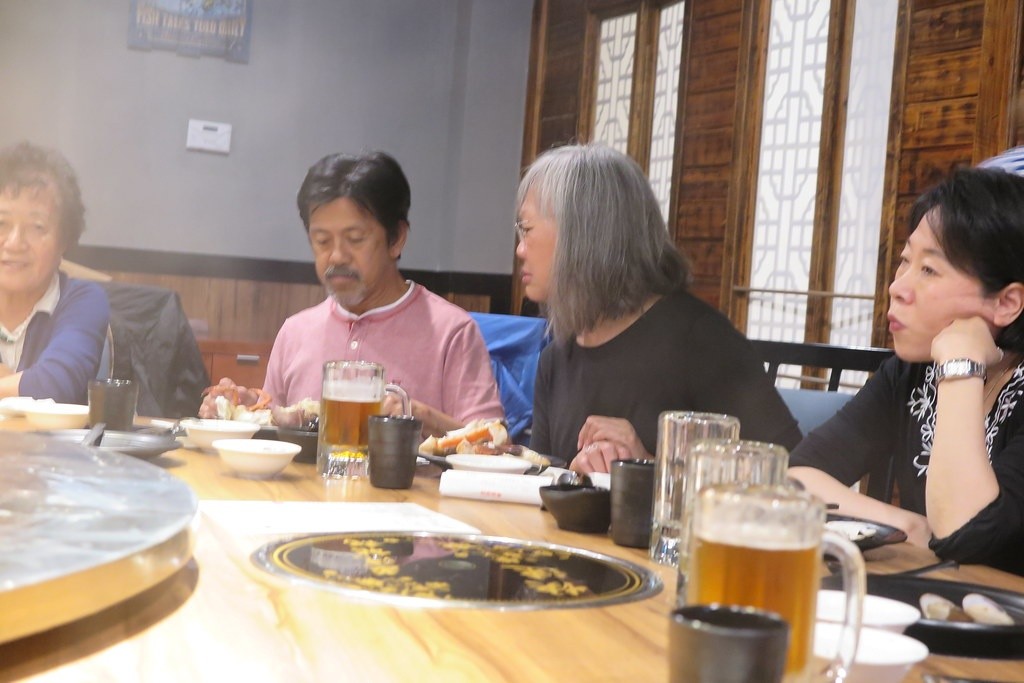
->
<box><xmin>935</xmin><ymin>359</ymin><xmax>988</xmax><ymax>384</ymax></box>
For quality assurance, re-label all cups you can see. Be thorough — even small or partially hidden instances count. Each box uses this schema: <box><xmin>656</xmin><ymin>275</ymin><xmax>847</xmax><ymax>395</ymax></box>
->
<box><xmin>86</xmin><ymin>376</ymin><xmax>140</xmax><ymax>432</ymax></box>
<box><xmin>683</xmin><ymin>483</ymin><xmax>867</xmax><ymax>683</ymax></box>
<box><xmin>315</xmin><ymin>358</ymin><xmax>412</xmax><ymax>481</ymax></box>
<box><xmin>678</xmin><ymin>436</ymin><xmax>788</xmax><ymax>520</ymax></box>
<box><xmin>666</xmin><ymin>602</ymin><xmax>791</xmax><ymax>683</ymax></box>
<box><xmin>647</xmin><ymin>410</ymin><xmax>741</xmax><ymax>569</ymax></box>
<box><xmin>366</xmin><ymin>414</ymin><xmax>423</xmax><ymax>490</ymax></box>
<box><xmin>609</xmin><ymin>457</ymin><xmax>655</xmax><ymax>550</ymax></box>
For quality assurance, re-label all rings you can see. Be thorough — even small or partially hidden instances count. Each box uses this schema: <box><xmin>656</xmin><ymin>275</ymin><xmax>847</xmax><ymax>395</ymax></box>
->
<box><xmin>997</xmin><ymin>346</ymin><xmax>1004</xmax><ymax>362</ymax></box>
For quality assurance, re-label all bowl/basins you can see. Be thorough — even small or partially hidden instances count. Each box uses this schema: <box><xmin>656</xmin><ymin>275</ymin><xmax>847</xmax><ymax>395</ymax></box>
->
<box><xmin>812</xmin><ymin>589</ymin><xmax>929</xmax><ymax>683</ymax></box>
<box><xmin>24</xmin><ymin>403</ymin><xmax>88</xmax><ymax>431</ymax></box>
<box><xmin>539</xmin><ymin>483</ymin><xmax>612</xmax><ymax>534</ymax></box>
<box><xmin>212</xmin><ymin>438</ymin><xmax>303</xmax><ymax>481</ymax></box>
<box><xmin>275</xmin><ymin>426</ymin><xmax>318</xmax><ymax>463</ymax></box>
<box><xmin>445</xmin><ymin>453</ymin><xmax>532</xmax><ymax>475</ymax></box>
<box><xmin>180</xmin><ymin>419</ymin><xmax>261</xmax><ymax>452</ymax></box>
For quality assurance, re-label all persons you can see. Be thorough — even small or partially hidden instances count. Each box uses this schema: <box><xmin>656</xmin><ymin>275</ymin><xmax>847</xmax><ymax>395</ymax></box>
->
<box><xmin>514</xmin><ymin>144</ymin><xmax>804</xmax><ymax>475</ymax></box>
<box><xmin>784</xmin><ymin>167</ymin><xmax>1024</xmax><ymax>576</ymax></box>
<box><xmin>199</xmin><ymin>151</ymin><xmax>508</xmax><ymax>441</ymax></box>
<box><xmin>0</xmin><ymin>141</ymin><xmax>109</xmax><ymax>405</ymax></box>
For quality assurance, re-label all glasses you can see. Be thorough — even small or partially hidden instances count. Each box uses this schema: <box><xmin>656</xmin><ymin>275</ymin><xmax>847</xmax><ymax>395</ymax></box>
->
<box><xmin>514</xmin><ymin>214</ymin><xmax>555</xmax><ymax>244</ymax></box>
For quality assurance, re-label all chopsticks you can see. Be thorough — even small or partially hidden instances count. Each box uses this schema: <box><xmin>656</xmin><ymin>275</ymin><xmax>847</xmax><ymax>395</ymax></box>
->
<box><xmin>80</xmin><ymin>422</ymin><xmax>107</xmax><ymax>446</ymax></box>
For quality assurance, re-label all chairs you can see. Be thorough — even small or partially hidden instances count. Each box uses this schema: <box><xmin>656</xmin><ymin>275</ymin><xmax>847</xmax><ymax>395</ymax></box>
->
<box><xmin>466</xmin><ymin>311</ymin><xmax>549</xmax><ymax>448</ymax></box>
<box><xmin>749</xmin><ymin>341</ymin><xmax>896</xmax><ymax>508</ymax></box>
<box><xmin>95</xmin><ymin>281</ymin><xmax>210</xmax><ymax>420</ymax></box>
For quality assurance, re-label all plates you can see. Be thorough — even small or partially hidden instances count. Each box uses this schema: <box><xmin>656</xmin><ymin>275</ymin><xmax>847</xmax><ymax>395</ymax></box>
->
<box><xmin>416</xmin><ymin>452</ymin><xmax>567</xmax><ymax>477</ymax></box>
<box><xmin>822</xmin><ymin>513</ymin><xmax>907</xmax><ymax>560</ymax></box>
<box><xmin>818</xmin><ymin>573</ymin><xmax>1024</xmax><ymax>659</ymax></box>
<box><xmin>32</xmin><ymin>429</ymin><xmax>183</xmax><ymax>457</ymax></box>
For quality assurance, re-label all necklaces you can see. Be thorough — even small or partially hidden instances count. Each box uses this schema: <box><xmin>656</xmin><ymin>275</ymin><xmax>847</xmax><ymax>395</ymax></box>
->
<box><xmin>984</xmin><ymin>366</ymin><xmax>1012</xmax><ymax>398</ymax></box>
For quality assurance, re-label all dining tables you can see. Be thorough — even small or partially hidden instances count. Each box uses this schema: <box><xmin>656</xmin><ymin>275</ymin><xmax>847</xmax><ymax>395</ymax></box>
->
<box><xmin>1</xmin><ymin>401</ymin><xmax>1024</xmax><ymax>683</ymax></box>
